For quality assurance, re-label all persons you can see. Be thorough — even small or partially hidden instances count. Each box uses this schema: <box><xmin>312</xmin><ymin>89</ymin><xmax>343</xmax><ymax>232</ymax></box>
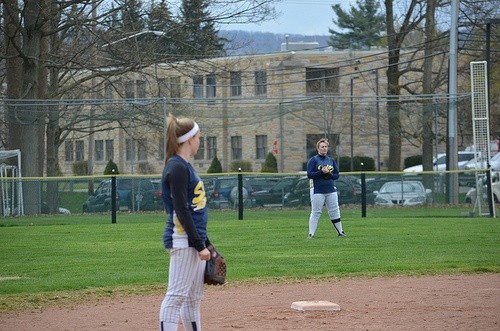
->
<box><xmin>159</xmin><ymin>113</ymin><xmax>216</xmax><ymax>331</ymax></box>
<box><xmin>306</xmin><ymin>138</ymin><xmax>346</xmax><ymax>239</ymax></box>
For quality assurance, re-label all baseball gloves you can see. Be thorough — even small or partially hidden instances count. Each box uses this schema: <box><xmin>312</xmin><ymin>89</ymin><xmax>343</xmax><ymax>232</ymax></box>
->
<box><xmin>203</xmin><ymin>249</ymin><xmax>226</xmax><ymax>285</ymax></box>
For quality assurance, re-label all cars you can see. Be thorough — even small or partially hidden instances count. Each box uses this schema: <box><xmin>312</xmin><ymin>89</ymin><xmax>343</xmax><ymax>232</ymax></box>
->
<box><xmin>372</xmin><ymin>181</ymin><xmax>433</xmax><ymax>209</ymax></box>
<box><xmin>465</xmin><ymin>171</ymin><xmax>500</xmax><ymax>204</ymax></box>
<box><xmin>253</xmin><ymin>176</ymin><xmax>297</xmax><ymax>204</ymax></box>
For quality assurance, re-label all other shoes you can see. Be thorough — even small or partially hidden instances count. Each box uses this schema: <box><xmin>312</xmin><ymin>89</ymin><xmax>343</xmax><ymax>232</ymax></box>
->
<box><xmin>306</xmin><ymin>234</ymin><xmax>315</xmax><ymax>240</ymax></box>
<box><xmin>337</xmin><ymin>231</ymin><xmax>347</xmax><ymax>237</ymax></box>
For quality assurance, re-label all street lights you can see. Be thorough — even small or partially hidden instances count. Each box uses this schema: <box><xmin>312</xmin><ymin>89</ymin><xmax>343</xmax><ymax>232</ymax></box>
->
<box><xmin>86</xmin><ymin>30</ymin><xmax>167</xmax><ymax>195</ymax></box>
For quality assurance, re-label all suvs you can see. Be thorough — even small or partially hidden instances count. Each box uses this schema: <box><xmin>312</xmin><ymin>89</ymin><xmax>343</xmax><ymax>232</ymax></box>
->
<box><xmin>152</xmin><ymin>180</ymin><xmax>166</xmax><ymax>211</ymax></box>
<box><xmin>205</xmin><ymin>177</ymin><xmax>256</xmax><ymax>209</ymax></box>
<box><xmin>283</xmin><ymin>176</ymin><xmax>358</xmax><ymax>208</ymax></box>
<box><xmin>404</xmin><ymin>151</ymin><xmax>481</xmax><ymax>173</ymax></box>
<box><xmin>83</xmin><ymin>177</ymin><xmax>157</xmax><ymax>213</ymax></box>
